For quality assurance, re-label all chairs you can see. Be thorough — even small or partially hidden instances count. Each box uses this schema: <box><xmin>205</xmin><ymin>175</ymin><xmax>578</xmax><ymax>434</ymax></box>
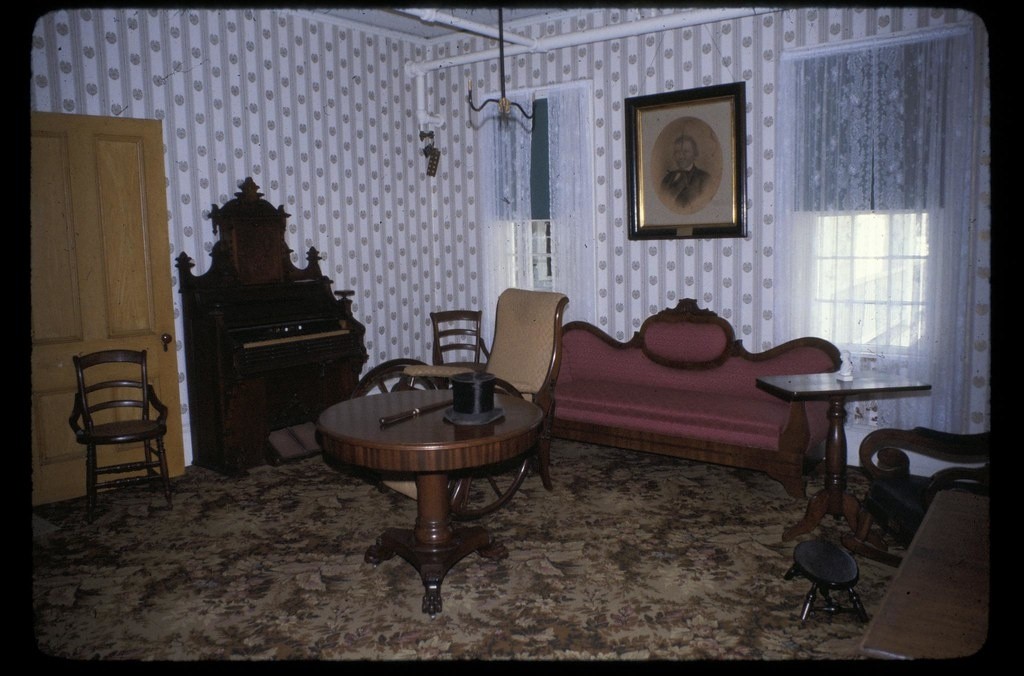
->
<box><xmin>70</xmin><ymin>349</ymin><xmax>174</xmax><ymax>524</ymax></box>
<box><xmin>429</xmin><ymin>309</ymin><xmax>490</xmax><ymax>377</ymax></box>
<box><xmin>843</xmin><ymin>426</ymin><xmax>990</xmax><ymax>571</ymax></box>
<box><xmin>345</xmin><ymin>287</ymin><xmax>570</xmax><ymax>517</ymax></box>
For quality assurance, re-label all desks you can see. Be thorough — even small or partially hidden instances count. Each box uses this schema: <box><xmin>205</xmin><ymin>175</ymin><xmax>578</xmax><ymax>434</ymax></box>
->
<box><xmin>861</xmin><ymin>491</ymin><xmax>991</xmax><ymax>659</ymax></box>
<box><xmin>317</xmin><ymin>390</ymin><xmax>545</xmax><ymax>615</ymax></box>
<box><xmin>755</xmin><ymin>372</ymin><xmax>932</xmax><ymax>541</ymax></box>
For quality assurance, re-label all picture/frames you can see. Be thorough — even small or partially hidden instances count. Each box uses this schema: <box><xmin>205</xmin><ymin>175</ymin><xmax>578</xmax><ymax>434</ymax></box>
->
<box><xmin>625</xmin><ymin>82</ymin><xmax>748</xmax><ymax>240</ymax></box>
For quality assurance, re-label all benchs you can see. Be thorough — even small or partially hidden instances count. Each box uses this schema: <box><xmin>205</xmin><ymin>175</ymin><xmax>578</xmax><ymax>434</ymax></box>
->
<box><xmin>537</xmin><ymin>297</ymin><xmax>852</xmax><ymax>500</ymax></box>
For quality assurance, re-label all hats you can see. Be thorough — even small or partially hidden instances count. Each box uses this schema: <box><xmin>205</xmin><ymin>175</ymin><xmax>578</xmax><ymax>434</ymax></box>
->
<box><xmin>443</xmin><ymin>372</ymin><xmax>504</xmax><ymax>426</ymax></box>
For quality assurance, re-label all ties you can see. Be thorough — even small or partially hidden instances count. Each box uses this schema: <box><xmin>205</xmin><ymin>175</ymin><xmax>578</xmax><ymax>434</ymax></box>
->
<box><xmin>672</xmin><ymin>172</ymin><xmax>685</xmax><ymax>187</ymax></box>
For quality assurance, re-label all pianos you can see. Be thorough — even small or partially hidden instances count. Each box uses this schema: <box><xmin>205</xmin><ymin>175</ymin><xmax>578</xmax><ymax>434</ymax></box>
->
<box><xmin>181</xmin><ymin>283</ymin><xmax>370</xmax><ymax>477</ymax></box>
